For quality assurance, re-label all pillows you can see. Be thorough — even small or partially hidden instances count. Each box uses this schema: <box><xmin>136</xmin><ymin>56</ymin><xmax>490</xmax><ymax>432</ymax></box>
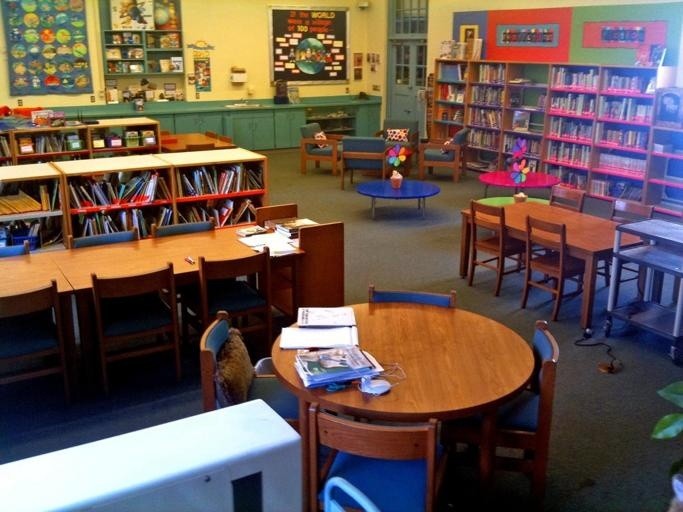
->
<box><xmin>315</xmin><ymin>131</ymin><xmax>328</xmax><ymax>148</ymax></box>
<box><xmin>440</xmin><ymin>139</ymin><xmax>453</xmax><ymax>154</ymax></box>
<box><xmin>386</xmin><ymin>128</ymin><xmax>410</xmax><ymax>142</ymax></box>
<box><xmin>215</xmin><ymin>328</ymin><xmax>256</xmax><ymax>406</ymax></box>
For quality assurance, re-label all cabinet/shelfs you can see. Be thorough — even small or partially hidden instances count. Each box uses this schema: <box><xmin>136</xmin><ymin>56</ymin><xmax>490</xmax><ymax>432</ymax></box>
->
<box><xmin>586</xmin><ymin>65</ymin><xmax>675</xmax><ymax>207</ymax></box>
<box><xmin>463</xmin><ymin>59</ymin><xmax>518</xmax><ymax>173</ymax></box>
<box><xmin>431</xmin><ymin>57</ymin><xmax>468</xmax><ymax>167</ymax></box>
<box><xmin>0</xmin><ymin>117</ymin><xmax>163</xmax><ymax>166</ymax></box>
<box><xmin>155</xmin><ymin>116</ymin><xmax>175</xmax><ymax>134</ymax></box>
<box><xmin>97</xmin><ymin>0</ymin><xmax>185</xmax><ymax>78</ymax></box>
<box><xmin>175</xmin><ymin>111</ymin><xmax>224</xmax><ymax>135</ymax></box>
<box><xmin>543</xmin><ymin>63</ymin><xmax>601</xmax><ymax>195</ymax></box>
<box><xmin>224</xmin><ymin>109</ymin><xmax>276</xmax><ymax>151</ymax></box>
<box><xmin>644</xmin><ymin>127</ymin><xmax>683</xmax><ymax>217</ymax></box>
<box><xmin>356</xmin><ymin>104</ymin><xmax>383</xmax><ymax>139</ymax></box>
<box><xmin>275</xmin><ymin>109</ymin><xmax>307</xmax><ymax>150</ymax></box>
<box><xmin>1</xmin><ymin>147</ymin><xmax>268</xmax><ymax>255</ymax></box>
<box><xmin>308</xmin><ymin>106</ymin><xmax>355</xmax><ymax>134</ymax></box>
<box><xmin>498</xmin><ymin>59</ymin><xmax>550</xmax><ymax>174</ymax></box>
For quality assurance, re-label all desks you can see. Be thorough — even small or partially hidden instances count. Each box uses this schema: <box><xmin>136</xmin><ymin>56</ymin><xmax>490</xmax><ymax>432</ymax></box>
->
<box><xmin>355</xmin><ymin>180</ymin><xmax>441</xmax><ymax>219</ymax></box>
<box><xmin>361</xmin><ymin>147</ymin><xmax>413</xmax><ymax>176</ymax></box>
<box><xmin>476</xmin><ymin>196</ymin><xmax>550</xmax><ymax>206</ymax></box>
<box><xmin>479</xmin><ymin>170</ymin><xmax>561</xmax><ymax>199</ymax></box>
<box><xmin>159</xmin><ymin>132</ymin><xmax>238</xmax><ymax>153</ymax></box>
<box><xmin>271</xmin><ymin>302</ymin><xmax>535</xmax><ymax>475</ymax></box>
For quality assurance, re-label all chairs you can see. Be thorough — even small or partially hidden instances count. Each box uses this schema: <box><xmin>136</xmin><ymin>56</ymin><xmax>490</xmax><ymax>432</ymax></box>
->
<box><xmin>518</xmin><ymin>185</ymin><xmax>586</xmax><ymax>281</ymax></box>
<box><xmin>309</xmin><ymin>403</ymin><xmax>449</xmax><ymax>511</ymax></box>
<box><xmin>597</xmin><ymin>200</ymin><xmax>655</xmax><ymax>296</ymax></box>
<box><xmin>183</xmin><ymin>246</ymin><xmax>274</xmax><ymax>358</ymax></box>
<box><xmin>160</xmin><ymin>131</ymin><xmax>169</xmax><ymax>136</ymax></box>
<box><xmin>199</xmin><ymin>311</ymin><xmax>300</xmax><ymax>432</ymax></box>
<box><xmin>459</xmin><ymin>319</ymin><xmax>560</xmax><ymax>503</ymax></box>
<box><xmin>367</xmin><ymin>283</ymin><xmax>457</xmax><ymax>308</ymax></box>
<box><xmin>1</xmin><ymin>279</ymin><xmax>74</xmax><ymax>407</ymax></box>
<box><xmin>375</xmin><ymin>119</ymin><xmax>421</xmax><ymax>165</ymax></box>
<box><xmin>339</xmin><ymin>136</ymin><xmax>390</xmax><ymax>190</ymax></box>
<box><xmin>419</xmin><ymin>127</ymin><xmax>471</xmax><ymax>183</ymax></box>
<box><xmin>186</xmin><ymin>143</ymin><xmax>215</xmax><ymax>151</ymax></box>
<box><xmin>521</xmin><ymin>214</ymin><xmax>585</xmax><ymax>321</ymax></box>
<box><xmin>206</xmin><ymin>130</ymin><xmax>217</xmax><ymax>138</ymax></box>
<box><xmin>151</xmin><ymin>216</ymin><xmax>215</xmax><ymax>237</ymax></box>
<box><xmin>0</xmin><ymin>240</ymin><xmax>30</xmax><ymax>255</ymax></box>
<box><xmin>467</xmin><ymin>198</ymin><xmax>526</xmax><ymax>298</ymax></box>
<box><xmin>299</xmin><ymin>123</ymin><xmax>343</xmax><ymax>176</ymax></box>
<box><xmin>219</xmin><ymin>135</ymin><xmax>233</xmax><ymax>143</ymax></box>
<box><xmin>66</xmin><ymin>227</ymin><xmax>139</xmax><ymax>248</ymax></box>
<box><xmin>89</xmin><ymin>261</ymin><xmax>184</xmax><ymax>394</ymax></box>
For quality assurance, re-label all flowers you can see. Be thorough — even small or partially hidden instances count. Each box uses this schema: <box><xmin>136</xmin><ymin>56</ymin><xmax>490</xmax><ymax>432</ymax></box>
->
<box><xmin>510</xmin><ymin>158</ymin><xmax>531</xmax><ymax>194</ymax></box>
<box><xmin>388</xmin><ymin>144</ymin><xmax>407</xmax><ymax>174</ymax></box>
<box><xmin>512</xmin><ymin>137</ymin><xmax>527</xmax><ymax>158</ymax></box>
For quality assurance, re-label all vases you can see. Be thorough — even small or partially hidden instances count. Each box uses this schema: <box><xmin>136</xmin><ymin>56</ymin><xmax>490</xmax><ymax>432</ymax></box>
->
<box><xmin>391</xmin><ymin>177</ymin><xmax>403</xmax><ymax>189</ymax></box>
<box><xmin>513</xmin><ymin>193</ymin><xmax>528</xmax><ymax>202</ymax></box>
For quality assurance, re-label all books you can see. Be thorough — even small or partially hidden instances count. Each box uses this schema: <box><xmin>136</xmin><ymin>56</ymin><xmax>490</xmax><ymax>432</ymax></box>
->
<box><xmin>91</xmin><ymin>129</ymin><xmax>158</xmax><ymax>149</ymax></box>
<box><xmin>598</xmin><ymin>152</ymin><xmax>647</xmax><ymax>177</ymax></box>
<box><xmin>546</xmin><ymin>139</ymin><xmax>590</xmax><ymax>168</ymax></box>
<box><xmin>467</xmin><ymin>127</ymin><xmax>501</xmax><ymax>151</ymax></box>
<box><xmin>594</xmin><ymin>122</ymin><xmax>650</xmax><ymax>151</ymax></box>
<box><xmin>548</xmin><ymin>115</ymin><xmax>593</xmax><ymax>142</ymax></box>
<box><xmin>67</xmin><ymin>169</ymin><xmax>172</xmax><ymax>209</ymax></box>
<box><xmin>511</xmin><ymin>110</ymin><xmax>531</xmax><ymax>133</ymax></box>
<box><xmin>508</xmin><ymin>86</ymin><xmax>526</xmax><ymax>108</ymax></box>
<box><xmin>278</xmin><ymin>306</ymin><xmax>384</xmax><ymax>391</ymax></box>
<box><xmin>550</xmin><ymin>92</ymin><xmax>597</xmax><ymax>115</ymax></box>
<box><xmin>107</xmin><ymin>60</ymin><xmax>146</xmax><ymax>74</ymax></box>
<box><xmin>0</xmin><ymin>216</ymin><xmax>63</xmax><ymax>252</ymax></box>
<box><xmin>14</xmin><ymin>130</ymin><xmax>86</xmax><ymax>156</ymax></box>
<box><xmin>437</xmin><ymin>61</ymin><xmax>469</xmax><ymax>82</ymax></box>
<box><xmin>0</xmin><ymin>135</ymin><xmax>13</xmax><ymax>159</ymax></box>
<box><xmin>18</xmin><ymin>153</ymin><xmax>86</xmax><ymax>166</ymax></box>
<box><xmin>467</xmin><ymin>107</ymin><xmax>502</xmax><ymax>129</ymax></box>
<box><xmin>146</xmin><ymin>32</ymin><xmax>182</xmax><ymax>49</ymax></box>
<box><xmin>526</xmin><ymin>159</ymin><xmax>539</xmax><ymax>172</ymax></box>
<box><xmin>589</xmin><ymin>178</ymin><xmax>643</xmax><ymax>202</ymax></box>
<box><xmin>602</xmin><ymin>68</ymin><xmax>656</xmax><ymax>95</ymax></box>
<box><xmin>536</xmin><ymin>93</ymin><xmax>547</xmax><ymax>111</ymax></box>
<box><xmin>111</xmin><ymin>31</ymin><xmax>141</xmax><ymax>45</ymax></box>
<box><xmin>508</xmin><ymin>78</ymin><xmax>532</xmax><ymax>84</ymax></box>
<box><xmin>634</xmin><ymin>43</ymin><xmax>667</xmax><ymax>68</ymax></box>
<box><xmin>175</xmin><ymin>162</ymin><xmax>264</xmax><ymax>199</ymax></box>
<box><xmin>470</xmin><ymin>85</ymin><xmax>505</xmax><ymax>107</ymax></box>
<box><xmin>435</xmin><ymin>102</ymin><xmax>465</xmax><ymax>123</ymax></box>
<box><xmin>235</xmin><ymin>216</ymin><xmax>319</xmax><ymax>258</ymax></box>
<box><xmin>30</xmin><ymin>109</ymin><xmax>83</xmax><ymax>127</ymax></box>
<box><xmin>439</xmin><ymin>38</ymin><xmax>483</xmax><ymax>61</ymax></box>
<box><xmin>74</xmin><ymin>204</ymin><xmax>175</xmax><ymax>241</ymax></box>
<box><xmin>0</xmin><ymin>177</ymin><xmax>63</xmax><ymax>215</ymax></box>
<box><xmin>654</xmin><ymin>86</ymin><xmax>683</xmax><ymax>130</ymax></box>
<box><xmin>550</xmin><ymin>66</ymin><xmax>599</xmax><ymax>90</ymax></box>
<box><xmin>478</xmin><ymin>63</ymin><xmax>506</xmax><ymax>84</ymax></box>
<box><xmin>177</xmin><ymin>197</ymin><xmax>257</xmax><ymax>229</ymax></box>
<box><xmin>437</xmin><ymin>82</ymin><xmax>466</xmax><ymax>104</ymax></box>
<box><xmin>542</xmin><ymin>162</ymin><xmax>588</xmax><ymax>190</ymax></box>
<box><xmin>502</xmin><ymin>133</ymin><xmax>541</xmax><ymax>158</ymax></box>
<box><xmin>276</xmin><ymin>79</ymin><xmax>301</xmax><ymax>104</ymax></box>
<box><xmin>105</xmin><ymin>78</ymin><xmax>183</xmax><ymax>112</ymax></box>
<box><xmin>598</xmin><ymin>95</ymin><xmax>653</xmax><ymax>124</ymax></box>
<box><xmin>105</xmin><ymin>46</ymin><xmax>146</xmax><ymax>60</ymax></box>
<box><xmin>147</xmin><ymin>55</ymin><xmax>184</xmax><ymax>73</ymax></box>
<box><xmin>92</xmin><ymin>150</ymin><xmax>133</xmax><ymax>159</ymax></box>
